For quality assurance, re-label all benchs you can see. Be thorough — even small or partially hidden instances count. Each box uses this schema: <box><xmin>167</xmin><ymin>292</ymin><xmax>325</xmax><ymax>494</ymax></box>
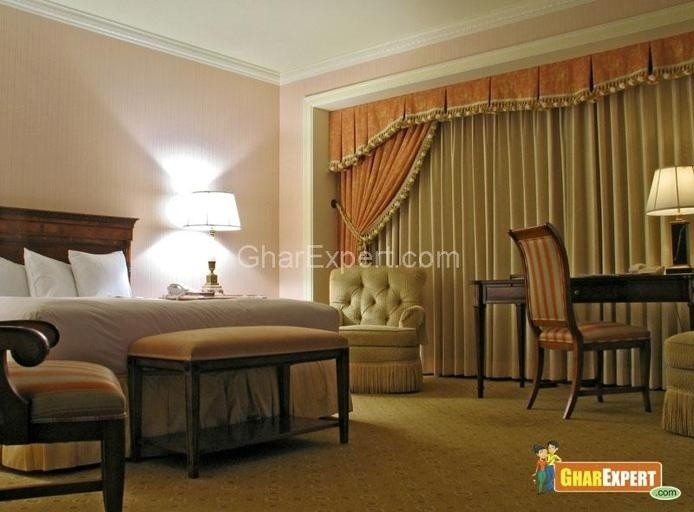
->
<box><xmin>126</xmin><ymin>323</ymin><xmax>349</xmax><ymax>479</ymax></box>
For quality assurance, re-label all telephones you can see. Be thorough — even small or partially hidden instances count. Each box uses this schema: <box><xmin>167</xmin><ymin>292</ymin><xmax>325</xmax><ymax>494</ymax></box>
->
<box><xmin>628</xmin><ymin>263</ymin><xmax>665</xmax><ymax>276</ymax></box>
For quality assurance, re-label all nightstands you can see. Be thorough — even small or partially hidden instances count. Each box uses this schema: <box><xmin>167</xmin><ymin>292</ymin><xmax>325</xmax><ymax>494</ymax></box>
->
<box><xmin>158</xmin><ymin>293</ymin><xmax>264</xmax><ymax>301</ymax></box>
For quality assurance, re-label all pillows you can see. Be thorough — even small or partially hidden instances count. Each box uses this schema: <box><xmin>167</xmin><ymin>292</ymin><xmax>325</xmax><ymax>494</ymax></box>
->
<box><xmin>0</xmin><ymin>256</ymin><xmax>30</xmax><ymax>297</ymax></box>
<box><xmin>23</xmin><ymin>245</ymin><xmax>78</xmax><ymax>298</ymax></box>
<box><xmin>67</xmin><ymin>247</ymin><xmax>131</xmax><ymax>297</ymax></box>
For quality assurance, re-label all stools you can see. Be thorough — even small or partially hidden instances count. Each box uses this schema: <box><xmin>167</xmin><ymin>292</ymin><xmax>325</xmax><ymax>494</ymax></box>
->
<box><xmin>661</xmin><ymin>330</ymin><xmax>694</xmax><ymax>437</ymax></box>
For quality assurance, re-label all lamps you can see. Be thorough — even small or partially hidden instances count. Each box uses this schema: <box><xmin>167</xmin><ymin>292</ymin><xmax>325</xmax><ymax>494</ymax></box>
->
<box><xmin>644</xmin><ymin>165</ymin><xmax>694</xmax><ymax>273</ymax></box>
<box><xmin>183</xmin><ymin>190</ymin><xmax>241</xmax><ymax>293</ymax></box>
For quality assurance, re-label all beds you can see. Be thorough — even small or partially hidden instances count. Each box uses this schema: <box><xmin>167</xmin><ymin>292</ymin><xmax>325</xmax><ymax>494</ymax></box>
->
<box><xmin>0</xmin><ymin>205</ymin><xmax>342</xmax><ymax>473</ymax></box>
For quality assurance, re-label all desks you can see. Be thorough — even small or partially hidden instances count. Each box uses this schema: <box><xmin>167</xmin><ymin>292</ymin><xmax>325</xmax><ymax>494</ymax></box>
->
<box><xmin>468</xmin><ymin>271</ymin><xmax>694</xmax><ymax>400</ymax></box>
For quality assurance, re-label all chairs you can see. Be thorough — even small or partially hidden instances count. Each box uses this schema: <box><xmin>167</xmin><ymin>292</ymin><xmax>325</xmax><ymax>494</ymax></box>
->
<box><xmin>0</xmin><ymin>319</ymin><xmax>126</xmax><ymax>512</ymax></box>
<box><xmin>329</xmin><ymin>266</ymin><xmax>429</xmax><ymax>393</ymax></box>
<box><xmin>507</xmin><ymin>222</ymin><xmax>652</xmax><ymax>420</ymax></box>
